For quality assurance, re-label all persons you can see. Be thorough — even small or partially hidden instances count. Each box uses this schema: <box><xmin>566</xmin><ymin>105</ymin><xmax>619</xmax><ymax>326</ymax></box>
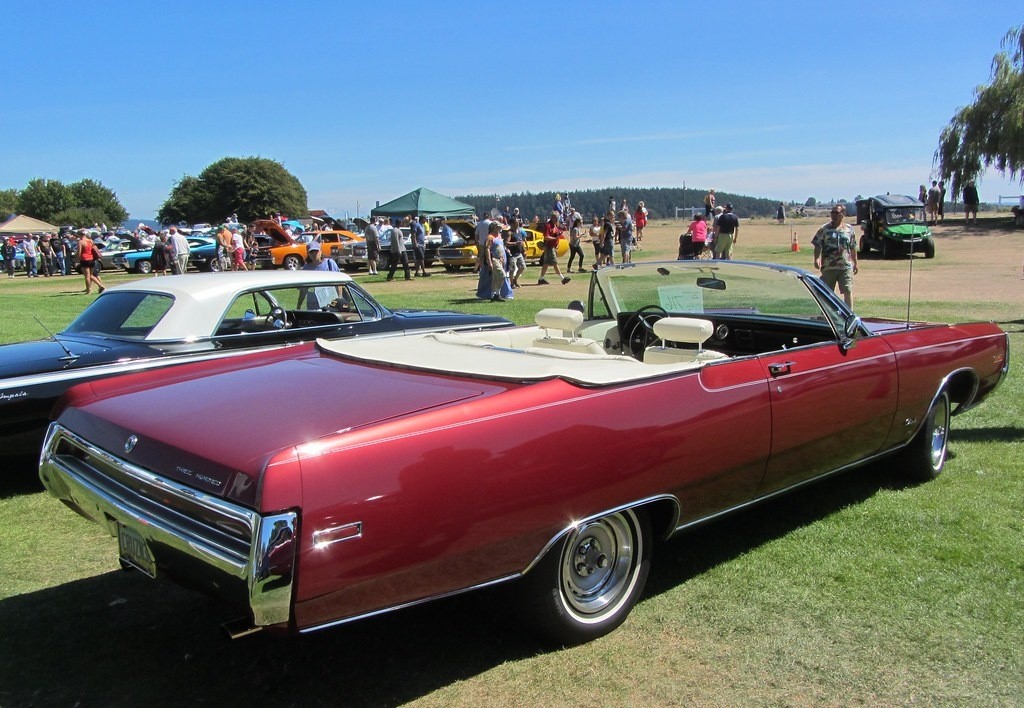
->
<box><xmin>411</xmin><ymin>214</ymin><xmax>432</xmax><ymax>276</ymax></box>
<box><xmin>366</xmin><ymin>217</ymin><xmax>382</xmax><ymax>275</ymax></box>
<box><xmin>963</xmin><ymin>179</ymin><xmax>980</xmax><ymax>224</ymax></box>
<box><xmin>438</xmin><ymin>218</ymin><xmax>452</xmax><ymax>245</ymax></box>
<box><xmin>216</xmin><ymin>224</ymin><xmax>258</xmax><ymax>271</ymax></box>
<box><xmin>78</xmin><ymin>230</ymin><xmax>105</xmax><ymax>295</ymax></box>
<box><xmin>1</xmin><ymin>232</ymin><xmax>74</xmax><ymax>279</ymax></box>
<box><xmin>590</xmin><ymin>196</ymin><xmax>648</xmax><ymax>270</ymax></box>
<box><xmin>810</xmin><ymin>205</ymin><xmax>858</xmax><ymax>313</ymax></box>
<box><xmin>285</xmin><ymin>220</ymin><xmax>334</xmax><ymax>243</ymax></box>
<box><xmin>93</xmin><ymin>222</ymin><xmax>158</xmax><ymax>242</ymax></box>
<box><xmin>777</xmin><ymin>201</ymin><xmax>805</xmax><ymax>225</ymax></box>
<box><xmin>296</xmin><ymin>241</ymin><xmax>343</xmax><ymax>312</ymax></box>
<box><xmin>685</xmin><ymin>188</ymin><xmax>739</xmax><ymax>259</ymax></box>
<box><xmin>538</xmin><ymin>192</ymin><xmax>588</xmax><ymax>284</ymax></box>
<box><xmin>151</xmin><ymin>227</ymin><xmax>190</xmax><ymax>277</ymax></box>
<box><xmin>475</xmin><ymin>206</ymin><xmax>527</xmax><ymax>302</ymax></box>
<box><xmin>225</xmin><ymin>213</ymin><xmax>238</xmax><ymax>224</ymax></box>
<box><xmin>918</xmin><ymin>181</ymin><xmax>946</xmax><ymax>226</ymax></box>
<box><xmin>388</xmin><ymin>219</ymin><xmax>415</xmax><ymax>281</ymax></box>
<box><xmin>900</xmin><ymin>212</ymin><xmax>918</xmax><ymax>223</ymax></box>
<box><xmin>269</xmin><ymin>213</ymin><xmax>283</xmax><ymax>228</ymax></box>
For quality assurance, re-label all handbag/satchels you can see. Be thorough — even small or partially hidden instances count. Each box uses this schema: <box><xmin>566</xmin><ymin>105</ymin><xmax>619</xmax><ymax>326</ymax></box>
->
<box><xmin>90</xmin><ymin>240</ymin><xmax>102</xmax><ymax>260</ymax></box>
<box><xmin>703</xmin><ymin>195</ymin><xmax>710</xmax><ymax>205</ymax></box>
<box><xmin>632</xmin><ymin>237</ymin><xmax>637</xmax><ymax>245</ymax></box>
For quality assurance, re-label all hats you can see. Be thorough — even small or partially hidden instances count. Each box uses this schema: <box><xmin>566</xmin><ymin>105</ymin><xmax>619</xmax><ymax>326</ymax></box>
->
<box><xmin>489</xmin><ymin>221</ymin><xmax>504</xmax><ymax>233</ymax></box>
<box><xmin>218</xmin><ymin>225</ymin><xmax>224</xmax><ymax>230</ymax></box>
<box><xmin>170</xmin><ymin>227</ymin><xmax>177</xmax><ymax>234</ymax></box>
<box><xmin>307</xmin><ymin>241</ymin><xmax>320</xmax><ymax>251</ymax></box>
<box><xmin>421</xmin><ymin>214</ymin><xmax>426</xmax><ymax>219</ymax></box>
<box><xmin>727</xmin><ymin>203</ymin><xmax>733</xmax><ymax>208</ymax></box>
<box><xmin>4</xmin><ymin>237</ymin><xmax>9</xmax><ymax>241</ymax></box>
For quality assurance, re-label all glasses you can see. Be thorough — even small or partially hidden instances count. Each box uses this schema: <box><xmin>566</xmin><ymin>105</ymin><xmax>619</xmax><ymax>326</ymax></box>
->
<box><xmin>831</xmin><ymin>211</ymin><xmax>843</xmax><ymax>214</ymax></box>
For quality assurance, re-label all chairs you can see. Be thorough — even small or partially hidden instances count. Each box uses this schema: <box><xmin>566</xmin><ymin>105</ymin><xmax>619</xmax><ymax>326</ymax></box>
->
<box><xmin>643</xmin><ymin>317</ymin><xmax>729</xmax><ymax>364</ymax></box>
<box><xmin>532</xmin><ymin>309</ymin><xmax>609</xmax><ymax>355</ymax></box>
<box><xmin>217</xmin><ymin>323</ymin><xmax>277</xmax><ymax>334</ymax></box>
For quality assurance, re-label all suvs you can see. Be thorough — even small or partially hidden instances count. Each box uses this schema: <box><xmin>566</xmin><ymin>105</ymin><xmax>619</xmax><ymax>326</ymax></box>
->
<box><xmin>855</xmin><ymin>192</ymin><xmax>935</xmax><ymax>259</ymax></box>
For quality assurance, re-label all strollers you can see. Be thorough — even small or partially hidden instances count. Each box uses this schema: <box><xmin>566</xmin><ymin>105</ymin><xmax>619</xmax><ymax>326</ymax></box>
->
<box><xmin>675</xmin><ymin>232</ymin><xmax>696</xmax><ymax>261</ymax></box>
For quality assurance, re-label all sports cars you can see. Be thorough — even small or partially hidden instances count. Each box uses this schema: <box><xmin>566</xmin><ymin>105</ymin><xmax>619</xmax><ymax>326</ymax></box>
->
<box><xmin>36</xmin><ymin>256</ymin><xmax>1012</xmax><ymax>639</ymax></box>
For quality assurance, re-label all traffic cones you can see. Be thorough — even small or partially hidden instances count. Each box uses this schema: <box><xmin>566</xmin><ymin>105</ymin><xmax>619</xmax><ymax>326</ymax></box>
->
<box><xmin>791</xmin><ymin>232</ymin><xmax>801</xmax><ymax>252</ymax></box>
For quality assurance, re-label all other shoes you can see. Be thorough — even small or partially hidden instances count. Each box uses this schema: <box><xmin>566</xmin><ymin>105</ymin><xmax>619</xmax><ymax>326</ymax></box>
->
<box><xmin>367</xmin><ymin>270</ymin><xmax>432</xmax><ymax>283</ymax></box>
<box><xmin>489</xmin><ymin>261</ymin><xmax>614</xmax><ymax>302</ymax></box>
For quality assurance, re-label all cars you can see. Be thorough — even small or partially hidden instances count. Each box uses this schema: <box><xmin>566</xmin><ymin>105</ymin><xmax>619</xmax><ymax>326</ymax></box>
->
<box><xmin>0</xmin><ymin>269</ymin><xmax>517</xmax><ymax>463</ymax></box>
<box><xmin>438</xmin><ymin>218</ymin><xmax>570</xmax><ymax>273</ymax></box>
<box><xmin>1</xmin><ymin>209</ymin><xmax>464</xmax><ymax>279</ymax></box>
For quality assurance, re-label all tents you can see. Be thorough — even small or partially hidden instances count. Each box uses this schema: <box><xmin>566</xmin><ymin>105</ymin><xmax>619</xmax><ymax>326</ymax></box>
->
<box><xmin>371</xmin><ymin>187</ymin><xmax>475</xmax><ymax>218</ymax></box>
<box><xmin>0</xmin><ymin>214</ymin><xmax>60</xmax><ymax>231</ymax></box>
